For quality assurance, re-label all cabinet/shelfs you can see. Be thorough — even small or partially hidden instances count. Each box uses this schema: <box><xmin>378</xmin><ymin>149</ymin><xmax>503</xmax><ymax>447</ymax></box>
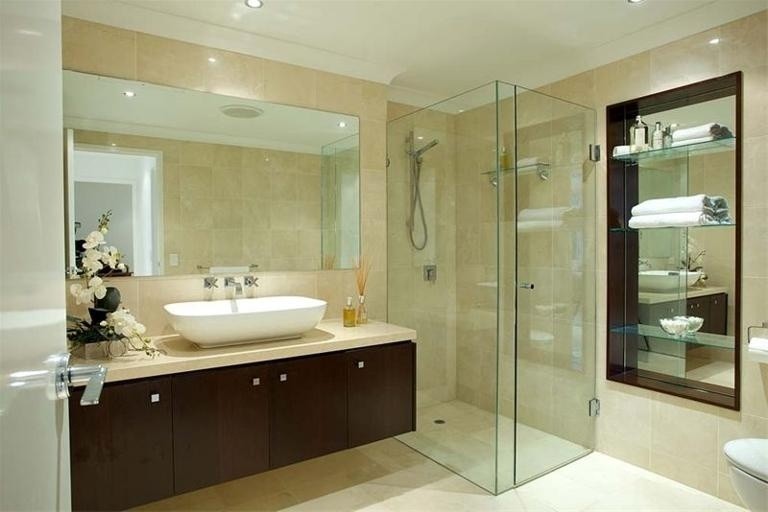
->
<box><xmin>173</xmin><ymin>349</ymin><xmax>348</xmax><ymax>497</ymax></box>
<box><xmin>610</xmin><ymin>135</ymin><xmax>735</xmax><ymax>349</ymax></box>
<box><xmin>68</xmin><ymin>374</ymin><xmax>174</xmax><ymax>512</ymax></box>
<box><xmin>638</xmin><ymin>301</ymin><xmax>678</xmax><ymax>352</ymax></box>
<box><xmin>345</xmin><ymin>341</ymin><xmax>416</xmax><ymax>452</ymax></box>
<box><xmin>709</xmin><ymin>292</ymin><xmax>728</xmax><ymax>336</ymax></box>
<box><xmin>680</xmin><ymin>295</ymin><xmax>709</xmax><ymax>349</ymax></box>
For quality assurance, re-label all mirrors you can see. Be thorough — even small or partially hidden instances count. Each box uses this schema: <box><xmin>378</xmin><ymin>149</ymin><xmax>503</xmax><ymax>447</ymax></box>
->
<box><xmin>63</xmin><ymin>68</ymin><xmax>360</xmax><ymax>282</ymax></box>
<box><xmin>638</xmin><ymin>166</ymin><xmax>674</xmax><ymax>259</ymax></box>
<box><xmin>625</xmin><ymin>86</ymin><xmax>737</xmax><ymax>397</ymax></box>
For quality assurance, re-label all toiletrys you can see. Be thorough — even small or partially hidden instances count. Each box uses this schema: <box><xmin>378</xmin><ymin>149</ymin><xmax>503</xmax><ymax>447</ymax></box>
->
<box><xmin>342</xmin><ymin>296</ymin><xmax>357</xmax><ymax>329</ymax></box>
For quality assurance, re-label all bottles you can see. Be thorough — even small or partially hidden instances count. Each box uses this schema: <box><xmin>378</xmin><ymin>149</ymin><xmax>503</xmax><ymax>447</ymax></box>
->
<box><xmin>653</xmin><ymin>123</ymin><xmax>663</xmax><ymax>149</ymax></box>
<box><xmin>663</xmin><ymin>127</ymin><xmax>672</xmax><ymax>148</ymax></box>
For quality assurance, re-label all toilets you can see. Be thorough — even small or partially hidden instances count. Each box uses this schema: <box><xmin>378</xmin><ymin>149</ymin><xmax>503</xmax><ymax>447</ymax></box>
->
<box><xmin>720</xmin><ymin>434</ymin><xmax>766</xmax><ymax>511</ymax></box>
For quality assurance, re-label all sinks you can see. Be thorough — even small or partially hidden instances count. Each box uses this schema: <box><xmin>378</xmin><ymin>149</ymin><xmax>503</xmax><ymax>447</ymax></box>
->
<box><xmin>163</xmin><ymin>295</ymin><xmax>328</xmax><ymax>349</ymax></box>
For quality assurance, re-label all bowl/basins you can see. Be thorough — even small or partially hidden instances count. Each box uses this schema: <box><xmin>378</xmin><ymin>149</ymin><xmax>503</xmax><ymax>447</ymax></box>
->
<box><xmin>658</xmin><ymin>318</ymin><xmax>689</xmax><ymax>335</ymax></box>
<box><xmin>674</xmin><ymin>313</ymin><xmax>704</xmax><ymax>336</ymax></box>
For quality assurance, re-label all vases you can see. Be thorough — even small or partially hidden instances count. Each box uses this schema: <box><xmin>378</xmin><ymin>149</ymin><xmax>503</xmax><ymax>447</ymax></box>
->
<box><xmin>356</xmin><ymin>295</ymin><xmax>369</xmax><ymax>324</ymax></box>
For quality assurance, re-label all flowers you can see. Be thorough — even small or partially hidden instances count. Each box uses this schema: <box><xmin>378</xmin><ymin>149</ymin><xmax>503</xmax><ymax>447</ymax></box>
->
<box><xmin>70</xmin><ymin>208</ymin><xmax>167</xmax><ymax>358</ymax></box>
<box><xmin>676</xmin><ymin>234</ymin><xmax>709</xmax><ymax>289</ymax></box>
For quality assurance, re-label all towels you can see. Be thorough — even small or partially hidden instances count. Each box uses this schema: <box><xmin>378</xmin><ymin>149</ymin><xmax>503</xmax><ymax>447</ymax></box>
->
<box><xmin>711</xmin><ymin>196</ymin><xmax>735</xmax><ymax>224</ymax></box>
<box><xmin>517</xmin><ymin>207</ymin><xmax>576</xmax><ymax>232</ymax></box>
<box><xmin>720</xmin><ymin>125</ymin><xmax>734</xmax><ymax>138</ymax></box>
<box><xmin>209</xmin><ymin>267</ymin><xmax>250</xmax><ymax>274</ymax></box>
<box><xmin>671</xmin><ymin>123</ymin><xmax>720</xmax><ymax>147</ymax></box>
<box><xmin>516</xmin><ymin>157</ymin><xmax>541</xmax><ymax>176</ymax></box>
<box><xmin>628</xmin><ymin>194</ymin><xmax>720</xmax><ymax>228</ymax></box>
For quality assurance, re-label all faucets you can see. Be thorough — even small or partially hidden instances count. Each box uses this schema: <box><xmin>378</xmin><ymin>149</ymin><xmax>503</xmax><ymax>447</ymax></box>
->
<box><xmin>222</xmin><ymin>276</ymin><xmax>244</xmax><ymax>297</ymax></box>
